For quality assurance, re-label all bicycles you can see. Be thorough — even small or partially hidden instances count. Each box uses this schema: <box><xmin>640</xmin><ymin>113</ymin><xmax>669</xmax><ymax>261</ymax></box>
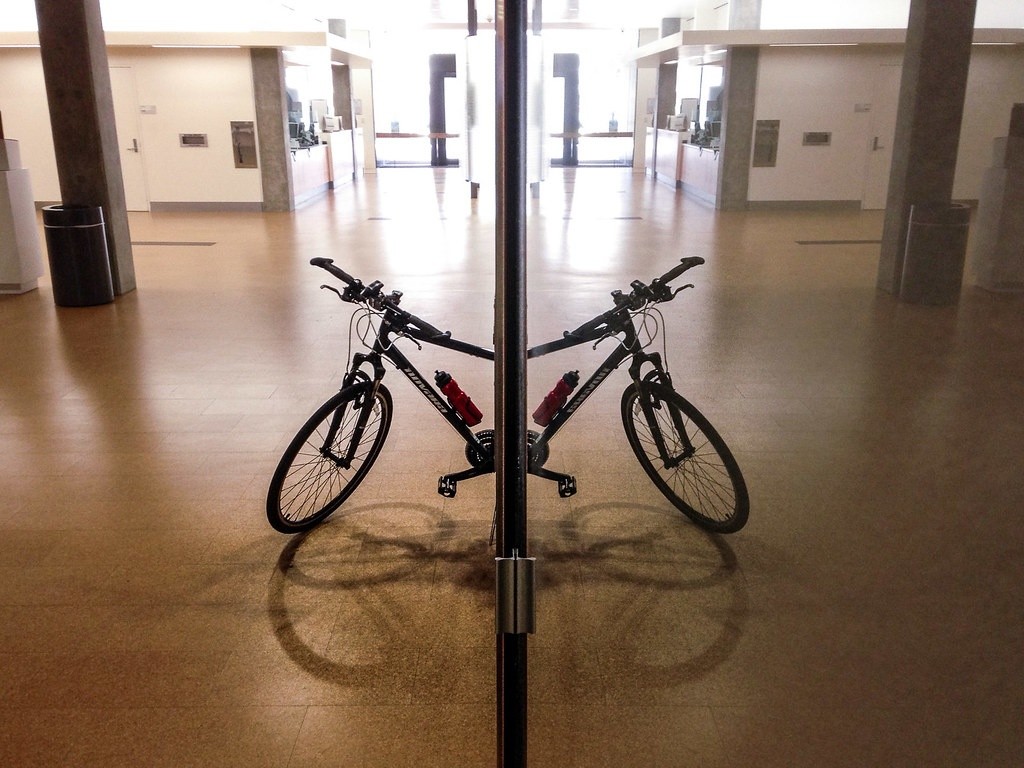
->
<box><xmin>265</xmin><ymin>256</ymin><xmax>496</xmax><ymax>536</ymax></box>
<box><xmin>528</xmin><ymin>256</ymin><xmax>750</xmax><ymax>536</ymax></box>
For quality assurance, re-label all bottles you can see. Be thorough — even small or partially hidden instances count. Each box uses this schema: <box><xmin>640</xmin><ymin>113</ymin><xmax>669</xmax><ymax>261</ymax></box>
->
<box><xmin>532</xmin><ymin>370</ymin><xmax>579</xmax><ymax>428</ymax></box>
<box><xmin>434</xmin><ymin>369</ymin><xmax>483</xmax><ymax>427</ymax></box>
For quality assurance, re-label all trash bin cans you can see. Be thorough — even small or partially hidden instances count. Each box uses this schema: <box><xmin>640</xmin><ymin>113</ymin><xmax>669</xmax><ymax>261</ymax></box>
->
<box><xmin>902</xmin><ymin>202</ymin><xmax>974</xmax><ymax>307</ymax></box>
<box><xmin>40</xmin><ymin>200</ymin><xmax>114</xmax><ymax>308</ymax></box>
